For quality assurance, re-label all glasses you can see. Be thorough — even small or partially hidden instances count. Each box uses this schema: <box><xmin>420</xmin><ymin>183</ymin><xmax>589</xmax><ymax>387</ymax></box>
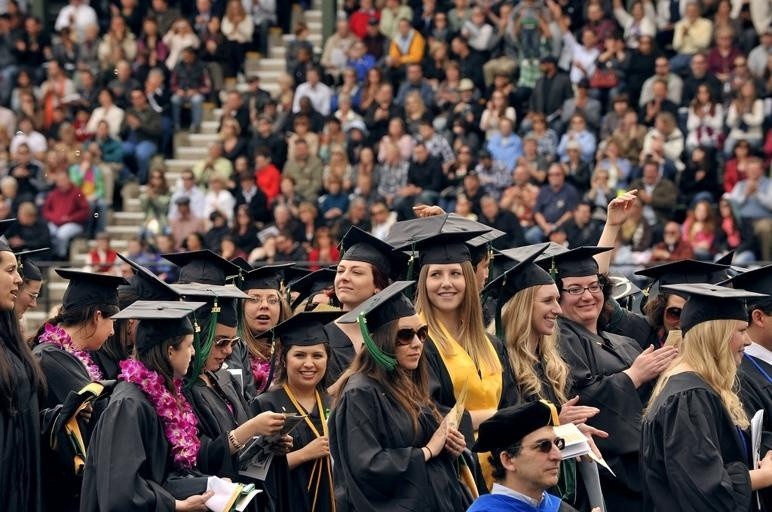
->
<box><xmin>20</xmin><ymin>286</ymin><xmax>40</xmax><ymax>301</ymax></box>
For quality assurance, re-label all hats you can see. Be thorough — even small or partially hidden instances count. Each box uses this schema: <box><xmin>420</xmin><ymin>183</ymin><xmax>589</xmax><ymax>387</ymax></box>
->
<box><xmin>470</xmin><ymin>402</ymin><xmax>554</xmax><ymax>454</ymax></box>
<box><xmin>335</xmin><ymin>212</ymin><xmax>616</xmax><ymax>347</ymax></box>
<box><xmin>0</xmin><ymin>234</ymin><xmax>13</xmax><ymax>252</ymax></box>
<box><xmin>55</xmin><ymin>247</ymin><xmax>335</xmax><ymax>345</ymax></box>
<box><xmin>633</xmin><ymin>250</ymin><xmax>772</xmax><ymax>339</ymax></box>
<box><xmin>13</xmin><ymin>247</ymin><xmax>50</xmax><ymax>281</ymax></box>
